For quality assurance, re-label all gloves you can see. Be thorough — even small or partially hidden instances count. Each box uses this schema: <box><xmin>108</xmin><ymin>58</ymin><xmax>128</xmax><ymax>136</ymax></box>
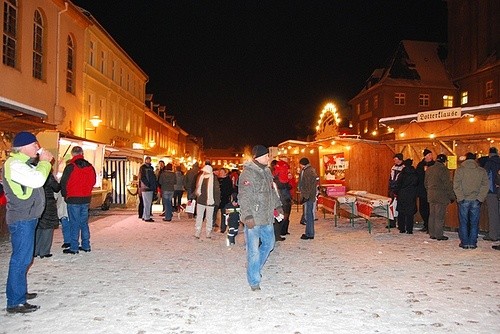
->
<box><xmin>276</xmin><ymin>206</ymin><xmax>284</xmax><ymax>214</ymax></box>
<box><xmin>475</xmin><ymin>199</ymin><xmax>479</xmax><ymax>201</ymax></box>
<box><xmin>245</xmin><ymin>215</ymin><xmax>255</xmax><ymax>228</ymax></box>
<box><xmin>462</xmin><ymin>199</ymin><xmax>464</xmax><ymax>203</ymax></box>
<box><xmin>187</xmin><ymin>200</ymin><xmax>191</xmax><ymax>205</ymax></box>
<box><xmin>448</xmin><ymin>196</ymin><xmax>455</xmax><ymax>203</ymax></box>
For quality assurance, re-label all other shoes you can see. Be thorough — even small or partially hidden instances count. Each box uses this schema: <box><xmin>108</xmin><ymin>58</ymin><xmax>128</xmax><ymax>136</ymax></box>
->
<box><xmin>140</xmin><ymin>209</ymin><xmax>319</xmax><ymax>244</ymax></box>
<box><xmin>386</xmin><ymin>224</ymin><xmax>426</xmax><ymax>234</ymax></box>
<box><xmin>483</xmin><ymin>237</ymin><xmax>498</xmax><ymax>241</ymax></box>
<box><xmin>492</xmin><ymin>244</ymin><xmax>500</xmax><ymax>250</ymax></box>
<box><xmin>252</xmin><ymin>284</ymin><xmax>260</xmax><ymax>291</ymax></box>
<box><xmin>470</xmin><ymin>245</ymin><xmax>477</xmax><ymax>248</ymax></box>
<box><xmin>438</xmin><ymin>236</ymin><xmax>448</xmax><ymax>240</ymax></box>
<box><xmin>33</xmin><ymin>242</ymin><xmax>90</xmax><ymax>258</ymax></box>
<box><xmin>459</xmin><ymin>243</ymin><xmax>468</xmax><ymax>249</ymax></box>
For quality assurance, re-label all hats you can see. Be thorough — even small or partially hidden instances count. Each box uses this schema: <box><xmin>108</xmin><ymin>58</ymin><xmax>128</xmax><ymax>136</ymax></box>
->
<box><xmin>395</xmin><ymin>153</ymin><xmax>403</xmax><ymax>160</ymax></box>
<box><xmin>436</xmin><ymin>154</ymin><xmax>447</xmax><ymax>162</ymax></box>
<box><xmin>466</xmin><ymin>152</ymin><xmax>474</xmax><ymax>160</ymax></box>
<box><xmin>423</xmin><ymin>148</ymin><xmax>431</xmax><ymax>156</ymax></box>
<box><xmin>252</xmin><ymin>145</ymin><xmax>268</xmax><ymax>159</ymax></box>
<box><xmin>489</xmin><ymin>147</ymin><xmax>497</xmax><ymax>153</ymax></box>
<box><xmin>13</xmin><ymin>132</ymin><xmax>37</xmax><ymax>147</ymax></box>
<box><xmin>201</xmin><ymin>165</ymin><xmax>212</xmax><ymax>174</ymax></box>
<box><xmin>299</xmin><ymin>158</ymin><xmax>310</xmax><ymax>166</ymax></box>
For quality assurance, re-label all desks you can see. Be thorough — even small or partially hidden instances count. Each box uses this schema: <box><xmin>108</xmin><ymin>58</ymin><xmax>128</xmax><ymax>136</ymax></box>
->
<box><xmin>318</xmin><ymin>194</ymin><xmax>356</xmax><ymax>227</ymax></box>
<box><xmin>347</xmin><ymin>191</ymin><xmax>394</xmax><ymax>234</ymax></box>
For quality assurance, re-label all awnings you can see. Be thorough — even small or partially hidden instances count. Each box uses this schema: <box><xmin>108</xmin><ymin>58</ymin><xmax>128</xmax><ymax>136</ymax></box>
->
<box><xmin>60</xmin><ymin>131</ymin><xmax>106</xmax><ymax>145</ymax></box>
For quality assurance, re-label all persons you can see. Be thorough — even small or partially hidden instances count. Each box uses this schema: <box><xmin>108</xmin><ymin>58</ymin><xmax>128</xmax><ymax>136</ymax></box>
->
<box><xmin>138</xmin><ymin>157</ymin><xmax>319</xmax><ymax>246</ymax></box>
<box><xmin>2</xmin><ymin>131</ymin><xmax>54</xmax><ymax>314</ymax></box>
<box><xmin>33</xmin><ymin>146</ymin><xmax>97</xmax><ymax>258</ymax></box>
<box><xmin>237</xmin><ymin>146</ymin><xmax>283</xmax><ymax>291</ymax></box>
<box><xmin>385</xmin><ymin>147</ymin><xmax>500</xmax><ymax>251</ymax></box>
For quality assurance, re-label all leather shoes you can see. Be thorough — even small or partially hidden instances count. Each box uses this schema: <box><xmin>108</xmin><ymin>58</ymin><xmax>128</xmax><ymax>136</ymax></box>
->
<box><xmin>26</xmin><ymin>293</ymin><xmax>37</xmax><ymax>299</ymax></box>
<box><xmin>7</xmin><ymin>301</ymin><xmax>38</xmax><ymax>313</ymax></box>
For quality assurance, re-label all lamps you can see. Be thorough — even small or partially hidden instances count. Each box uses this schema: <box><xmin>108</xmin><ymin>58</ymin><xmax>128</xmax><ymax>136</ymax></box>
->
<box><xmin>85</xmin><ymin>115</ymin><xmax>102</xmax><ymax>133</ymax></box>
